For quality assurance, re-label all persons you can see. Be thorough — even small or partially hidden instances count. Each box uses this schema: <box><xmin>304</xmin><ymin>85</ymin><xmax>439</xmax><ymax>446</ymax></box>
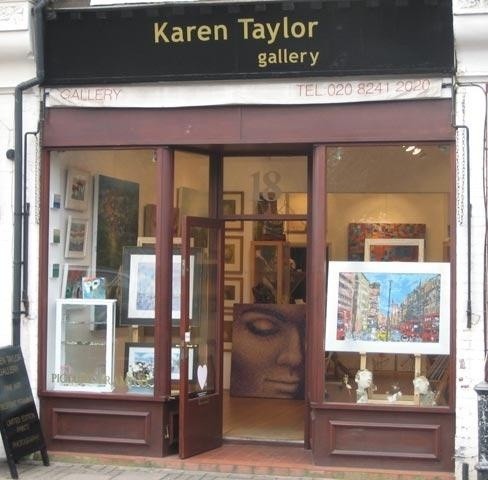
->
<box><xmin>231</xmin><ymin>303</ymin><xmax>306</xmax><ymax>400</ymax></box>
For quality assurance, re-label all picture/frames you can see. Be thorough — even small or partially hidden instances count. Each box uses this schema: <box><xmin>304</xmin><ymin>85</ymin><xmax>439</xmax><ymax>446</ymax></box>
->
<box><xmin>90</xmin><ymin>173</ymin><xmax>139</xmax><ymax>333</ymax></box>
<box><xmin>248</xmin><ymin>240</ymin><xmax>310</xmax><ymax>304</ymax></box>
<box><xmin>122</xmin><ymin>342</ymin><xmax>199</xmax><ymax>395</ymax></box>
<box><xmin>121</xmin><ymin>246</ymin><xmax>201</xmax><ymax>329</ymax></box>
<box><xmin>221</xmin><ymin>192</ymin><xmax>244</xmax><ymax>352</ymax></box>
<box><xmin>65</xmin><ymin>216</ymin><xmax>90</xmax><ymax>259</ymax></box>
<box><xmin>61</xmin><ymin>263</ymin><xmax>91</xmax><ymax>304</ymax></box>
<box><xmin>65</xmin><ymin>165</ymin><xmax>92</xmax><ymax>211</ymax></box>
<box><xmin>324</xmin><ymin>222</ymin><xmax>452</xmax><ymax>356</ymax></box>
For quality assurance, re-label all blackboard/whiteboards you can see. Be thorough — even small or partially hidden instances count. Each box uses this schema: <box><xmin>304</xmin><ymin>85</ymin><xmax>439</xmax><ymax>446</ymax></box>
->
<box><xmin>0</xmin><ymin>345</ymin><xmax>45</xmax><ymax>460</ymax></box>
